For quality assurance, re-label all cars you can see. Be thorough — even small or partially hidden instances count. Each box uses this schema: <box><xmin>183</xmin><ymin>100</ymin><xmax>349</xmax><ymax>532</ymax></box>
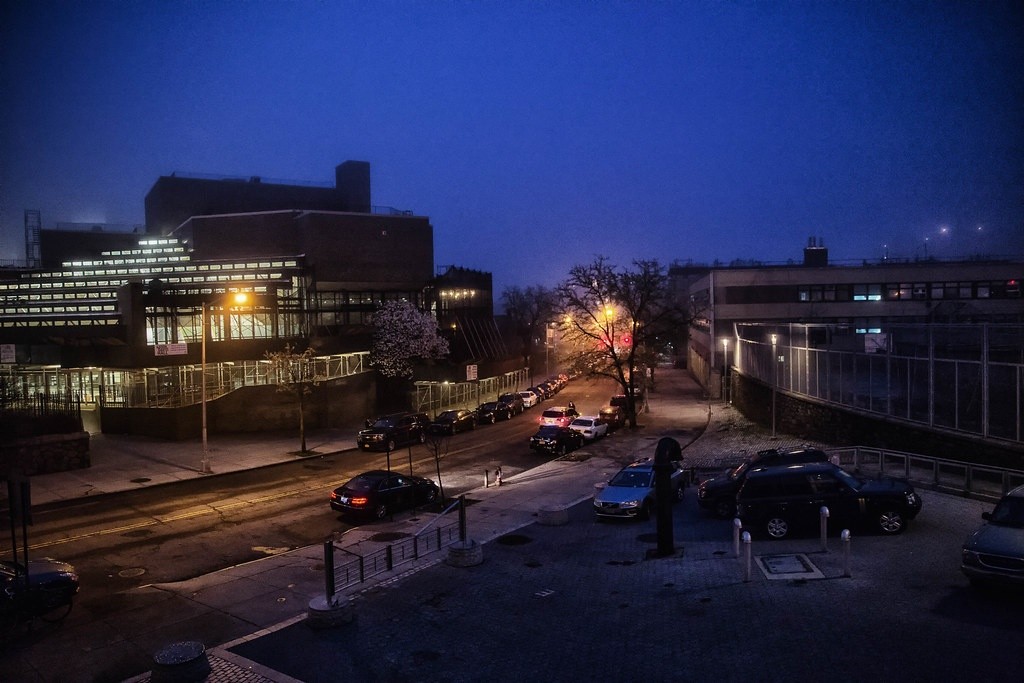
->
<box><xmin>475</xmin><ymin>401</ymin><xmax>512</xmax><ymax>424</ymax></box>
<box><xmin>0</xmin><ymin>557</ymin><xmax>81</xmax><ymax>629</ymax></box>
<box><xmin>599</xmin><ymin>405</ymin><xmax>625</xmax><ymax>427</ymax></box>
<box><xmin>527</xmin><ymin>368</ymin><xmax>582</xmax><ymax>403</ymax></box>
<box><xmin>431</xmin><ymin>408</ymin><xmax>478</xmax><ymax>437</ymax></box>
<box><xmin>516</xmin><ymin>390</ymin><xmax>537</xmax><ymax>408</ymax></box>
<box><xmin>330</xmin><ymin>468</ymin><xmax>439</xmax><ymax>519</ymax></box>
<box><xmin>566</xmin><ymin>416</ymin><xmax>609</xmax><ymax>441</ymax></box>
<box><xmin>529</xmin><ymin>426</ymin><xmax>586</xmax><ymax>458</ymax></box>
<box><xmin>961</xmin><ymin>483</ymin><xmax>1024</xmax><ymax>592</ymax></box>
<box><xmin>592</xmin><ymin>456</ymin><xmax>687</xmax><ymax>521</ymax></box>
<box><xmin>610</xmin><ymin>394</ymin><xmax>642</xmax><ymax>417</ymax></box>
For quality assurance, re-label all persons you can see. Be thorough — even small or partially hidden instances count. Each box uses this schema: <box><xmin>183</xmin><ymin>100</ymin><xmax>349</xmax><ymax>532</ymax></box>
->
<box><xmin>365</xmin><ymin>416</ymin><xmax>371</xmax><ymax>428</ymax></box>
<box><xmin>569</xmin><ymin>401</ymin><xmax>575</xmax><ymax>410</ymax></box>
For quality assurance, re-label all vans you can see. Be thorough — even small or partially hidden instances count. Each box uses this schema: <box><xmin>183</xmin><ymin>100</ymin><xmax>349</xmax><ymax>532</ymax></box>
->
<box><xmin>498</xmin><ymin>391</ymin><xmax>525</xmax><ymax>416</ymax></box>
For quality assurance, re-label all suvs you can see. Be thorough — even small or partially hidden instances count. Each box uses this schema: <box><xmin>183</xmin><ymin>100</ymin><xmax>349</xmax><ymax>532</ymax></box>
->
<box><xmin>736</xmin><ymin>461</ymin><xmax>923</xmax><ymax>541</ymax></box>
<box><xmin>357</xmin><ymin>411</ymin><xmax>432</xmax><ymax>453</ymax></box>
<box><xmin>538</xmin><ymin>405</ymin><xmax>581</xmax><ymax>432</ymax></box>
<box><xmin>696</xmin><ymin>448</ymin><xmax>827</xmax><ymax>521</ymax></box>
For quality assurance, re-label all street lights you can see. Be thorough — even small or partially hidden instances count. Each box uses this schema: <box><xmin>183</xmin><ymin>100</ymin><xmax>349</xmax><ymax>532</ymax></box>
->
<box><xmin>199</xmin><ymin>291</ymin><xmax>247</xmax><ymax>474</ymax></box>
<box><xmin>545</xmin><ymin>321</ymin><xmax>556</xmax><ymax>380</ymax></box>
